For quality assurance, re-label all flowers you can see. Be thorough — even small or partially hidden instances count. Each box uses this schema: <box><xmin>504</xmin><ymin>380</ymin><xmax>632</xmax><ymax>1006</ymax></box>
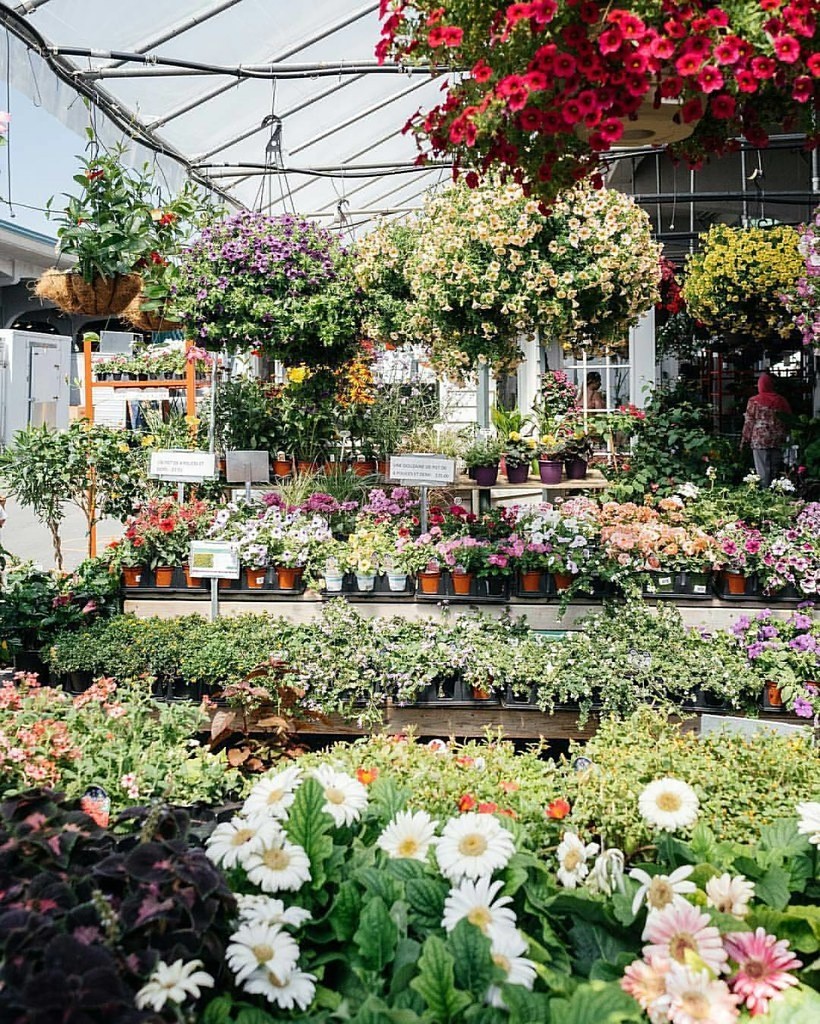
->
<box><xmin>0</xmin><ymin>466</ymin><xmax>820</xmax><ymax>1024</ymax></box>
<box><xmin>0</xmin><ymin>0</ymin><xmax>820</xmax><ymax>466</ymax></box>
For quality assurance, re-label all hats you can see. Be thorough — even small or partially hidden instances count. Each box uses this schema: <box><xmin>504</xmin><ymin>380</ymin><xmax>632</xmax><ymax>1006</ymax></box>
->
<box><xmin>587</xmin><ymin>372</ymin><xmax>601</xmax><ymax>387</ymax></box>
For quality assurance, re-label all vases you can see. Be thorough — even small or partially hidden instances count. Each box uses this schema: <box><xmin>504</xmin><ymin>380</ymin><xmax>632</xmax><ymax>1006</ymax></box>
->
<box><xmin>244</xmin><ymin>566</ymin><xmax>267</xmax><ymax>589</ymax></box>
<box><xmin>686</xmin><ymin>572</ymin><xmax>710</xmax><ymax>593</ymax></box>
<box><xmin>155</xmin><ymin>567</ymin><xmax>174</xmax><ymax>588</ymax></box>
<box><xmin>486</xmin><ymin>577</ymin><xmax>503</xmax><ymax>595</ymax></box>
<box><xmin>170</xmin><ymin>677</ymin><xmax>200</xmax><ymax>700</ymax></box>
<box><xmin>388</xmin><ymin>577</ymin><xmax>406</xmax><ymax>591</ymax></box>
<box><xmin>152</xmin><ymin>674</ymin><xmax>169</xmax><ymax>699</ymax></box>
<box><xmin>275</xmin><ymin>566</ymin><xmax>302</xmax><ymax>590</ymax></box>
<box><xmin>450</xmin><ymin>573</ymin><xmax>473</xmax><ymax>596</ymax></box>
<box><xmin>506</xmin><ymin>460</ymin><xmax>530</xmax><ymax>482</ymax></box>
<box><xmin>201</xmin><ymin>675</ymin><xmax>226</xmax><ymax>701</ymax></box>
<box><xmin>566</xmin><ymin>456</ymin><xmax>588</xmax><ymax>479</ymax></box>
<box><xmin>723</xmin><ymin>572</ymin><xmax>746</xmax><ymax>596</ymax></box>
<box><xmin>467</xmin><ymin>460</ymin><xmax>498</xmax><ymax>486</ymax></box>
<box><xmin>182</xmin><ymin>565</ymin><xmax>204</xmax><ymax>589</ymax></box>
<box><xmin>357</xmin><ymin>576</ymin><xmax>375</xmax><ymax>591</ymax></box>
<box><xmin>523</xmin><ymin>571</ymin><xmax>542</xmax><ymax>592</ymax></box>
<box><xmin>353</xmin><ymin>462</ymin><xmax>374</xmax><ymax>476</ymax></box>
<box><xmin>125</xmin><ymin>291</ymin><xmax>186</xmax><ymax>331</ymax></box>
<box><xmin>273</xmin><ymin>460</ymin><xmax>293</xmax><ymax>475</ymax></box>
<box><xmin>39</xmin><ymin>272</ymin><xmax>142</xmax><ymax>317</ymax></box>
<box><xmin>71</xmin><ymin>670</ymin><xmax>92</xmax><ymax>692</ymax></box>
<box><xmin>219</xmin><ymin>578</ymin><xmax>232</xmax><ymax>589</ymax></box>
<box><xmin>554</xmin><ymin>572</ymin><xmax>571</xmax><ymax>590</ymax></box>
<box><xmin>15</xmin><ymin>648</ymin><xmax>50</xmax><ymax>688</ymax></box>
<box><xmin>298</xmin><ymin>461</ymin><xmax>318</xmax><ymax>477</ymax></box>
<box><xmin>418</xmin><ymin>571</ymin><xmax>440</xmax><ymax>594</ymax></box>
<box><xmin>538</xmin><ymin>459</ymin><xmax>563</xmax><ymax>483</ymax></box>
<box><xmin>324</xmin><ymin>461</ymin><xmax>348</xmax><ymax>474</ymax></box>
<box><xmin>325</xmin><ymin>577</ymin><xmax>342</xmax><ymax>591</ymax></box>
<box><xmin>122</xmin><ymin>565</ymin><xmax>142</xmax><ymax>587</ymax></box>
<box><xmin>650</xmin><ymin>571</ymin><xmax>676</xmax><ymax>594</ymax></box>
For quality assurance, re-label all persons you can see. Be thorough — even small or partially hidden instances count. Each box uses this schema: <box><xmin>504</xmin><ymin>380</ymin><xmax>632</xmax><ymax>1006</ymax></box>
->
<box><xmin>740</xmin><ymin>375</ymin><xmax>792</xmax><ymax>488</ymax></box>
<box><xmin>579</xmin><ymin>372</ymin><xmax>604</xmax><ymax>418</ymax></box>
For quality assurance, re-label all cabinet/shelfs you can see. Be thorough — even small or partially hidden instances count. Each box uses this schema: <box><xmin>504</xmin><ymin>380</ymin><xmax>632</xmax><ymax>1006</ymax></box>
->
<box><xmin>83</xmin><ymin>340</ymin><xmax>212</xmax><ymax>558</ymax></box>
